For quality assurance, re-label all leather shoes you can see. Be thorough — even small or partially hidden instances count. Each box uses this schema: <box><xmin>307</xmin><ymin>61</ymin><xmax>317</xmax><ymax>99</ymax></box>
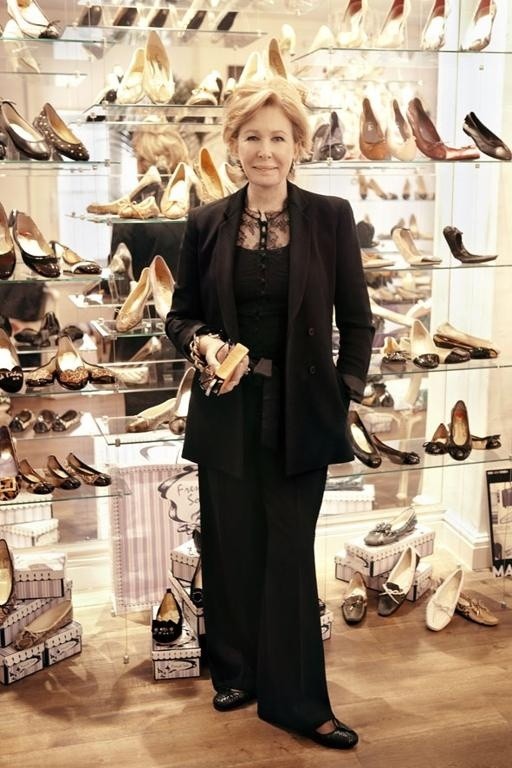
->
<box><xmin>200</xmin><ymin>71</ymin><xmax>223</xmax><ymax>104</ymax></box>
<box><xmin>152</xmin><ymin>588</ymin><xmax>182</xmax><ymax>644</ymax></box>
<box><xmin>411</xmin><ymin>318</ymin><xmax>441</xmax><ymax>369</ymax></box>
<box><xmin>20</xmin><ymin>459</ymin><xmax>55</xmax><ymax>495</ymax></box>
<box><xmin>87</xmin><ymin>197</ymin><xmax>132</xmax><ymax>214</ymax></box>
<box><xmin>347</xmin><ymin>411</ymin><xmax>382</xmax><ymax>468</ymax></box>
<box><xmin>367</xmin><ymin>178</ymin><xmax>388</xmax><ymax>200</ymax></box>
<box><xmin>13</xmin><ymin>600</ymin><xmax>73</xmax><ymax>650</ymax></box>
<box><xmin>437</xmin><ymin>321</ymin><xmax>504</xmax><ymax>354</ymax></box>
<box><xmin>117</xmin><ymin>195</ymin><xmax>160</xmax><ymax>220</ymax></box>
<box><xmin>320</xmin><ymin>111</ymin><xmax>347</xmax><ymax>160</ymax></box>
<box><xmin>32</xmin><ymin>409</ymin><xmax>56</xmax><ymax>433</ymax></box>
<box><xmin>369</xmin><ymin>432</ymin><xmax>421</xmax><ymax>465</ymax></box>
<box><xmin>413</xmin><ymin>390</ymin><xmax>428</xmax><ymax>411</ymax></box>
<box><xmin>417</xmin><ymin>175</ymin><xmax>427</xmax><ymax>200</ymax></box>
<box><xmin>193</xmin><ymin>147</ymin><xmax>224</xmax><ymax>205</ymax></box>
<box><xmin>358</xmin><ymin>174</ymin><xmax>367</xmax><ymax>199</ymax></box>
<box><xmin>43</xmin><ymin>455</ymin><xmax>82</xmax><ymax>490</ymax></box>
<box><xmin>34</xmin><ymin>102</ymin><xmax>90</xmax><ymax>161</ymax></box>
<box><xmin>447</xmin><ymin>422</ymin><xmax>502</xmax><ymax>449</ymax></box>
<box><xmin>51</xmin><ymin>409</ymin><xmax>81</xmax><ymax>432</ymax></box>
<box><xmin>81</xmin><ymin>359</ymin><xmax>116</xmax><ymax>384</ymax></box>
<box><xmin>462</xmin><ymin>111</ymin><xmax>512</xmax><ymax>161</ymax></box>
<box><xmin>160</xmin><ymin>161</ymin><xmax>192</xmax><ymax>219</ymax></box>
<box><xmin>377</xmin><ymin>545</ymin><xmax>421</xmax><ymax>616</ymax></box>
<box><xmin>224</xmin><ymin>76</ymin><xmax>238</xmax><ymax>99</ymax></box>
<box><xmin>212</xmin><ymin>687</ymin><xmax>256</xmax><ymax>711</ymax></box>
<box><xmin>13</xmin><ymin>327</ymin><xmax>50</xmax><ymax>346</ymax></box>
<box><xmin>433</xmin><ymin>334</ymin><xmax>498</xmax><ymax>360</ymax></box>
<box><xmin>38</xmin><ymin>310</ymin><xmax>60</xmax><ymax>335</ymax></box>
<box><xmin>387</xmin><ymin>193</ymin><xmax>398</xmax><ymax>200</ymax></box>
<box><xmin>8</xmin><ymin>407</ymin><xmax>35</xmax><ymax>432</ymax></box>
<box><xmin>341</xmin><ymin>571</ymin><xmax>368</xmax><ymax>623</ymax></box>
<box><xmin>63</xmin><ymin>452</ymin><xmax>112</xmax><ymax>487</ymax></box>
<box><xmin>191</xmin><ymin>525</ymin><xmax>202</xmax><ymax>553</ymax></box>
<box><xmin>300</xmin><ymin>123</ymin><xmax>329</xmax><ymax>163</ymax></box>
<box><xmin>190</xmin><ymin>553</ymin><xmax>204</xmax><ymax>607</ymax></box>
<box><xmin>364</xmin><ymin>507</ymin><xmax>417</xmax><ymax>545</ymax></box>
<box><xmin>318</xmin><ymin>598</ymin><xmax>326</xmax><ymax>616</ymax></box>
<box><xmin>0</xmin><ymin>538</ymin><xmax>18</xmax><ymax>627</ymax></box>
<box><xmin>400</xmin><ymin>336</ymin><xmax>470</xmax><ymax>364</ymax></box>
<box><xmin>447</xmin><ymin>399</ymin><xmax>473</xmax><ymax>461</ymax></box>
<box><xmin>126</xmin><ymin>398</ymin><xmax>177</xmax><ymax>432</ymax></box>
<box><xmin>386</xmin><ymin>97</ymin><xmax>417</xmax><ymax>162</ymax></box>
<box><xmin>422</xmin><ymin>423</ymin><xmax>451</xmax><ymax>455</ymax></box>
<box><xmin>0</xmin><ymin>425</ymin><xmax>22</xmax><ymax>501</ymax></box>
<box><xmin>372</xmin><ymin>382</ymin><xmax>394</xmax><ymax>407</ymax></box>
<box><xmin>425</xmin><ymin>568</ymin><xmax>465</xmax><ymax>632</ymax></box>
<box><xmin>361</xmin><ymin>385</ymin><xmax>381</xmax><ymax>408</ymax></box>
<box><xmin>55</xmin><ymin>325</ymin><xmax>84</xmax><ymax>345</ymax></box>
<box><xmin>296</xmin><ymin>716</ymin><xmax>359</xmax><ymax>748</ymax></box>
<box><xmin>443</xmin><ymin>145</ymin><xmax>480</xmax><ymax>161</ymax></box>
<box><xmin>405</xmin><ymin>96</ymin><xmax>447</xmax><ymax>160</ymax></box>
<box><xmin>431</xmin><ymin>576</ymin><xmax>499</xmax><ymax>625</ymax></box>
<box><xmin>380</xmin><ymin>335</ymin><xmax>407</xmax><ymax>364</ymax></box>
<box><xmin>0</xmin><ymin>201</ymin><xmax>16</xmax><ymax>280</ymax></box>
<box><xmin>12</xmin><ymin>211</ymin><xmax>61</xmax><ymax>278</ymax></box>
<box><xmin>10</xmin><ymin>365</ymin><xmax>24</xmax><ymax>392</ymax></box>
<box><xmin>169</xmin><ymin>367</ymin><xmax>196</xmax><ymax>436</ymax></box>
<box><xmin>359</xmin><ymin>97</ymin><xmax>392</xmax><ymax>160</ymax></box>
<box><xmin>175</xmin><ymin>90</ymin><xmax>217</xmax><ymax>122</ymax></box>
<box><xmin>0</xmin><ymin>368</ymin><xmax>10</xmax><ymax>392</ymax></box>
<box><xmin>25</xmin><ymin>355</ymin><xmax>55</xmax><ymax>386</ymax></box>
<box><xmin>0</xmin><ymin>101</ymin><xmax>52</xmax><ymax>160</ymax></box>
<box><xmin>402</xmin><ymin>179</ymin><xmax>411</xmax><ymax>200</ymax></box>
<box><xmin>49</xmin><ymin>240</ymin><xmax>103</xmax><ymax>275</ymax></box>
<box><xmin>55</xmin><ymin>334</ymin><xmax>89</xmax><ymax>390</ymax></box>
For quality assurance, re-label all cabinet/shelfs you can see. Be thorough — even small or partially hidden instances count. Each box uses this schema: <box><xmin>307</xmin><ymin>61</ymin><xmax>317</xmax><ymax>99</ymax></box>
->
<box><xmin>1</xmin><ymin>3</ymin><xmax>511</xmax><ymax>512</ymax></box>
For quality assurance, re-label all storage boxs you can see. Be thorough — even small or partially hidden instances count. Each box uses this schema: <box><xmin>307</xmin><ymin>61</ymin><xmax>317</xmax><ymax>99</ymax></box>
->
<box><xmin>0</xmin><ymin>645</ymin><xmax>46</xmax><ymax>685</ymax></box>
<box><xmin>0</xmin><ymin>494</ymin><xmax>53</xmax><ymax>523</ymax></box>
<box><xmin>171</xmin><ymin>538</ymin><xmax>201</xmax><ymax>584</ymax></box>
<box><xmin>167</xmin><ymin>573</ymin><xmax>205</xmax><ymax>638</ymax></box>
<box><xmin>314</xmin><ymin>483</ymin><xmax>377</xmax><ymax>517</ymax></box>
<box><xmin>148</xmin><ymin>606</ymin><xmax>201</xmax><ymax>680</ymax></box>
<box><xmin>318</xmin><ymin>604</ymin><xmax>331</xmax><ymax>644</ymax></box>
<box><xmin>35</xmin><ymin>617</ymin><xmax>84</xmax><ymax>669</ymax></box>
<box><xmin>3</xmin><ymin>552</ymin><xmax>65</xmax><ymax>600</ymax></box>
<box><xmin>346</xmin><ymin>525</ymin><xmax>434</xmax><ymax>578</ymax></box>
<box><xmin>5</xmin><ymin>517</ymin><xmax>60</xmax><ymax>549</ymax></box>
<box><xmin>0</xmin><ymin>580</ymin><xmax>74</xmax><ymax>646</ymax></box>
<box><xmin>335</xmin><ymin>558</ymin><xmax>432</xmax><ymax>602</ymax></box>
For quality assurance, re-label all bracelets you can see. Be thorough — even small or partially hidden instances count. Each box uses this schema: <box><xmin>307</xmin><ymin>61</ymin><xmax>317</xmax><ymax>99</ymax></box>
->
<box><xmin>188</xmin><ymin>330</ymin><xmax>220</xmax><ymax>374</ymax></box>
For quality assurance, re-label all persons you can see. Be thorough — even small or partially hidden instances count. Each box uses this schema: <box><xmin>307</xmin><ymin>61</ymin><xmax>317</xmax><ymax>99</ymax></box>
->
<box><xmin>165</xmin><ymin>87</ymin><xmax>376</xmax><ymax>751</ymax></box>
<box><xmin>101</xmin><ymin>125</ymin><xmax>208</xmax><ymax>416</ymax></box>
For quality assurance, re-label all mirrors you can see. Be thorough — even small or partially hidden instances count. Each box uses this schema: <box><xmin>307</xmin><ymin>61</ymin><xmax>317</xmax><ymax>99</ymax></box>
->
<box><xmin>484</xmin><ymin>468</ymin><xmax>512</xmax><ymax>578</ymax></box>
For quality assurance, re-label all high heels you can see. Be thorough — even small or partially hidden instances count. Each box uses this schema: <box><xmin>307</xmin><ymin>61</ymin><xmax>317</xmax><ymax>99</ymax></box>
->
<box><xmin>6</xmin><ymin>0</ymin><xmax>60</xmax><ymax>39</ymax></box>
<box><xmin>265</xmin><ymin>37</ymin><xmax>287</xmax><ymax>82</ymax></box>
<box><xmin>360</xmin><ymin>247</ymin><xmax>395</xmax><ymax>268</ymax></box>
<box><xmin>147</xmin><ymin>0</ymin><xmax>170</xmax><ymax>27</ymax></box>
<box><xmin>280</xmin><ymin>23</ymin><xmax>297</xmax><ymax>56</ymax></box>
<box><xmin>462</xmin><ymin>0</ymin><xmax>497</xmax><ymax>52</ymax></box>
<box><xmin>443</xmin><ymin>225</ymin><xmax>499</xmax><ymax>263</ymax></box>
<box><xmin>379</xmin><ymin>286</ymin><xmax>404</xmax><ymax>300</ymax></box>
<box><xmin>107</xmin><ymin>242</ymin><xmax>135</xmax><ymax>283</ymax></box>
<box><xmin>409</xmin><ymin>213</ymin><xmax>420</xmax><ymax>239</ymax></box>
<box><xmin>143</xmin><ymin>30</ymin><xmax>175</xmax><ymax>104</ymax></box>
<box><xmin>211</xmin><ymin>2</ymin><xmax>240</xmax><ymax>44</ymax></box>
<box><xmin>338</xmin><ymin>0</ymin><xmax>369</xmax><ymax>48</ymax></box>
<box><xmin>421</xmin><ymin>0</ymin><xmax>451</xmax><ymax>50</ymax></box>
<box><xmin>180</xmin><ymin>0</ymin><xmax>207</xmax><ymax>42</ymax></box>
<box><xmin>108</xmin><ymin>255</ymin><xmax>127</xmax><ymax>300</ymax></box>
<box><xmin>378</xmin><ymin>216</ymin><xmax>406</xmax><ymax>239</ymax></box>
<box><xmin>309</xmin><ymin>24</ymin><xmax>337</xmax><ymax>49</ymax></box>
<box><xmin>130</xmin><ymin>164</ymin><xmax>163</xmax><ymax>206</ymax></box>
<box><xmin>2</xmin><ymin>19</ymin><xmax>39</xmax><ymax>72</ymax></box>
<box><xmin>111</xmin><ymin>4</ymin><xmax>137</xmax><ymax>40</ymax></box>
<box><xmin>115</xmin><ymin>267</ymin><xmax>153</xmax><ymax>332</ymax></box>
<box><xmin>150</xmin><ymin>255</ymin><xmax>174</xmax><ymax>324</ymax></box>
<box><xmin>396</xmin><ymin>286</ymin><xmax>421</xmax><ymax>301</ymax></box>
<box><xmin>392</xmin><ymin>227</ymin><xmax>443</xmax><ymax>266</ymax></box>
<box><xmin>76</xmin><ymin>1</ymin><xmax>103</xmax><ymax>27</ymax></box>
<box><xmin>241</xmin><ymin>51</ymin><xmax>264</xmax><ymax>82</ymax></box>
<box><xmin>116</xmin><ymin>49</ymin><xmax>146</xmax><ymax>103</ymax></box>
<box><xmin>378</xmin><ymin>0</ymin><xmax>412</xmax><ymax>48</ymax></box>
